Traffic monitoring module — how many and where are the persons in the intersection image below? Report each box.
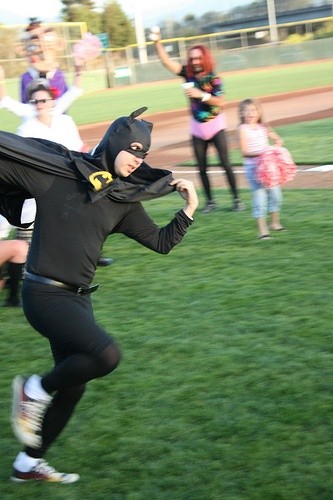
[0,48,115,309]
[150,26,244,213]
[0,108,199,483]
[238,99,285,241]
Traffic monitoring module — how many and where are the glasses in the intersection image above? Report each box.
[28,99,51,105]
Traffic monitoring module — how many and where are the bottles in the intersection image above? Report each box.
[148,25,159,40]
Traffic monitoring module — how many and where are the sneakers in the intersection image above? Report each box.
[10,372,49,449]
[9,457,80,485]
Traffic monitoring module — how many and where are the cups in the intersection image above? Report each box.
[182,81,194,91]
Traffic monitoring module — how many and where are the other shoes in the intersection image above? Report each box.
[271,223,283,231]
[232,200,245,212]
[256,231,271,240]
[200,202,218,214]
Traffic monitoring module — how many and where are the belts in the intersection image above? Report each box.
[21,271,100,295]
